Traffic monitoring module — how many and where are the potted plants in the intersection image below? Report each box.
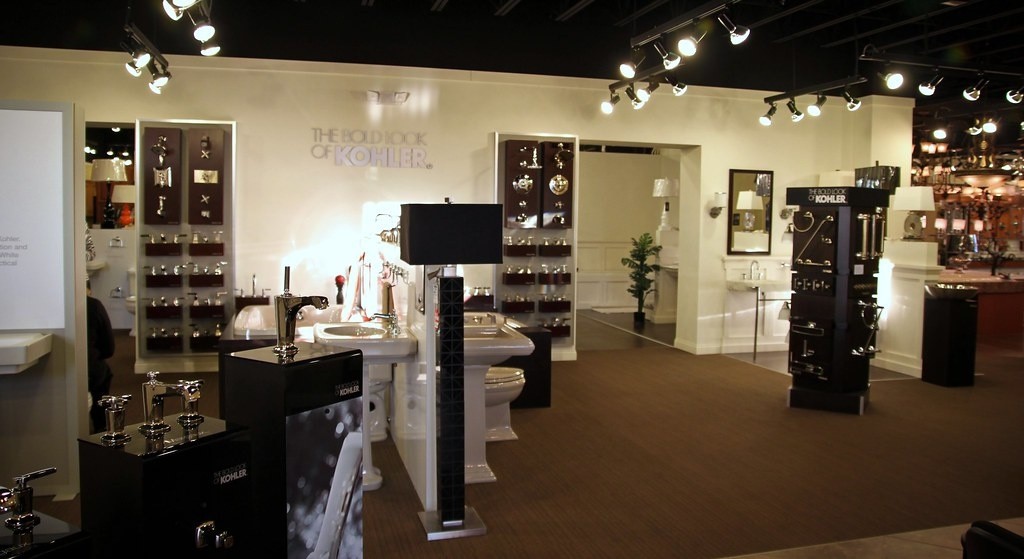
[621,233,662,329]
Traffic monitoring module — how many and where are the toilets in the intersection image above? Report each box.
[484,366,526,442]
[126,267,138,337]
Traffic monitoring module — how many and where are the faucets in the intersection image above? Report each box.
[749,259,759,280]
[503,234,574,330]
[138,228,225,341]
[251,272,259,298]
[141,368,201,431]
[273,264,332,360]
[369,309,400,335]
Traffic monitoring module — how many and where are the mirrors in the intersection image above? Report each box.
[727,169,773,255]
[86,120,136,232]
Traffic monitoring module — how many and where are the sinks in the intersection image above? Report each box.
[323,325,389,338]
[733,279,789,292]
[86,260,107,278]
[465,324,514,344]
[0,329,52,375]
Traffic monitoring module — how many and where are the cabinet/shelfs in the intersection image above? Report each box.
[133,119,236,375]
[489,132,579,361]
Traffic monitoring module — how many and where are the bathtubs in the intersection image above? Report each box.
[233,305,376,337]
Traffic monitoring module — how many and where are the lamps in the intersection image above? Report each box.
[600,60,690,114]
[366,90,410,106]
[777,302,791,320]
[163,0,220,56]
[910,140,1024,276]
[653,177,679,231]
[400,198,503,541]
[819,160,900,238]
[859,43,1024,104]
[620,1,751,79]
[756,174,771,232]
[152,136,218,217]
[912,107,1024,139]
[111,186,136,226]
[736,189,764,232]
[512,142,575,224]
[119,6,172,95]
[710,192,727,218]
[91,159,127,229]
[759,51,867,127]
[892,186,935,242]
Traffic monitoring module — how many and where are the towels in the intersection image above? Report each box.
[376,277,394,325]
[339,260,362,322]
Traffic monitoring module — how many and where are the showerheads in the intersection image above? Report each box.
[555,142,574,169]
[156,195,166,216]
[520,144,543,171]
